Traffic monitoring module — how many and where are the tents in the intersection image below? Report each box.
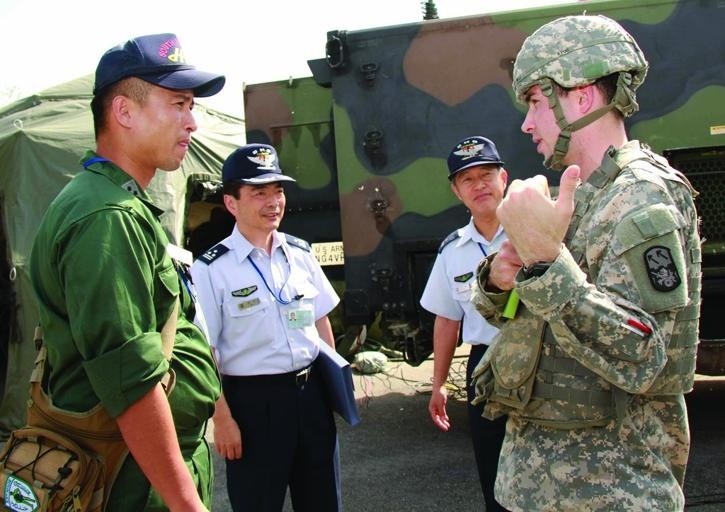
[0,72,248,442]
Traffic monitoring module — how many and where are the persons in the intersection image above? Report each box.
[419,136,511,511]
[27,33,226,511]
[187,144,343,511]
[470,14,704,511]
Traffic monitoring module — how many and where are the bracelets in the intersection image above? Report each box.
[429,377,447,383]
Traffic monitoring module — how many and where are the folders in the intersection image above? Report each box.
[318,337,362,426]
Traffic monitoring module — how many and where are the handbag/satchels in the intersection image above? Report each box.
[0,358,178,511]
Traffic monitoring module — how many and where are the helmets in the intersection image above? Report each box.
[511,13,649,105]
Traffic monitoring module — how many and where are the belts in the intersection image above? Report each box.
[293,367,313,389]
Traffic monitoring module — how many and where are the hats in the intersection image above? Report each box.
[94,34,226,98]
[446,136,504,179]
[222,143,297,186]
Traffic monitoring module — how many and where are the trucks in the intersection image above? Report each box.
[242,0,725,376]
